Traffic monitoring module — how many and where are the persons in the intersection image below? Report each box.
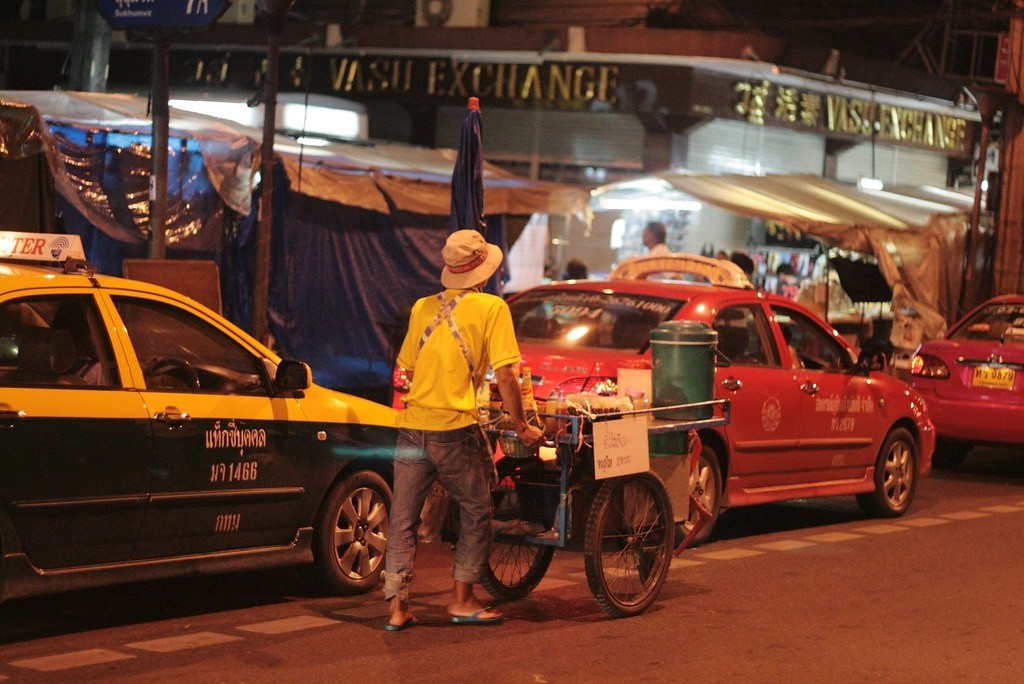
[776,266,801,299]
[643,221,685,280]
[564,257,588,280]
[51,300,107,386]
[757,323,805,369]
[378,230,549,631]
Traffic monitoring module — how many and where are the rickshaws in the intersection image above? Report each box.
[416,377,735,620]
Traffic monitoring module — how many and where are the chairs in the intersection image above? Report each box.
[716,325,749,358]
[612,311,651,349]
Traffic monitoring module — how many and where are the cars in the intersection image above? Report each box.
[0,227,412,604]
[438,277,939,552]
[909,291,1023,465]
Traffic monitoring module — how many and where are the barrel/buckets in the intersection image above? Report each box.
[648,319,732,419]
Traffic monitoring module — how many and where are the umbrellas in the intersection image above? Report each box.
[449,94,493,234]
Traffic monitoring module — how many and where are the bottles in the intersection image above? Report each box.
[514,368,651,443]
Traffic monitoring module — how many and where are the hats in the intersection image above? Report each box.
[440,229,504,289]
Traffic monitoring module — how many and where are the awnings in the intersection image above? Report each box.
[667,169,987,227]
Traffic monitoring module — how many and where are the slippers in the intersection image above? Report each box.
[384,613,418,631]
[452,606,506,622]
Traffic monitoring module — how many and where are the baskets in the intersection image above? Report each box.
[494,416,546,458]
[480,406,504,454]
